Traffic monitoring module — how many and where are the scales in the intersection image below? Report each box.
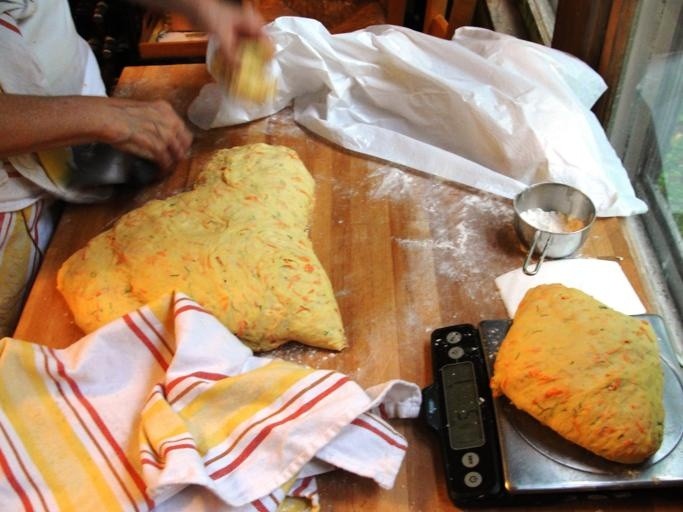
[419,313,683,509]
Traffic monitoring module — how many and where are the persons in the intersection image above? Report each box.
[0,0,279,343]
